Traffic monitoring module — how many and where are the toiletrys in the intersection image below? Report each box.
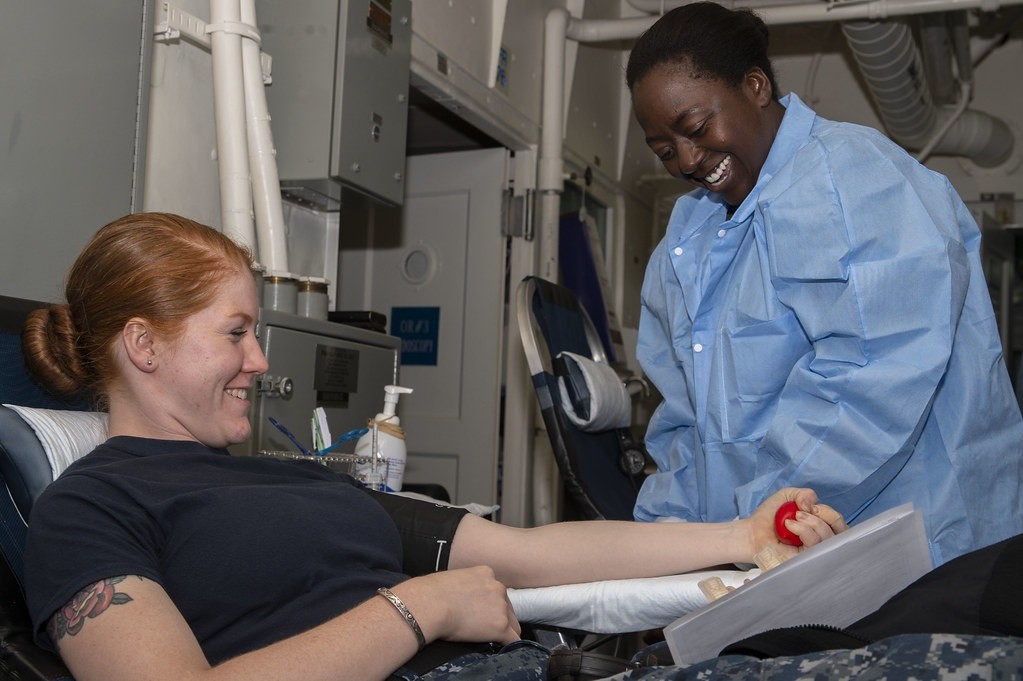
[350,384,414,493]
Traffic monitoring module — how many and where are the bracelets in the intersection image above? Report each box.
[377,588,425,651]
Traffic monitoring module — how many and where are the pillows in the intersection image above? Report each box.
[554,352,632,432]
[1,403,110,528]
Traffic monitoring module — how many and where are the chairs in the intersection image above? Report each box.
[515,276,652,521]
[0,296,114,681]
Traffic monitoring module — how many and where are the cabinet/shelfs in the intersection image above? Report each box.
[255,0,413,212]
[228,309,403,473]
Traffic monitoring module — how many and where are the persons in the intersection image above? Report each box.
[625,1,1023,659]
[21,213,850,681]
